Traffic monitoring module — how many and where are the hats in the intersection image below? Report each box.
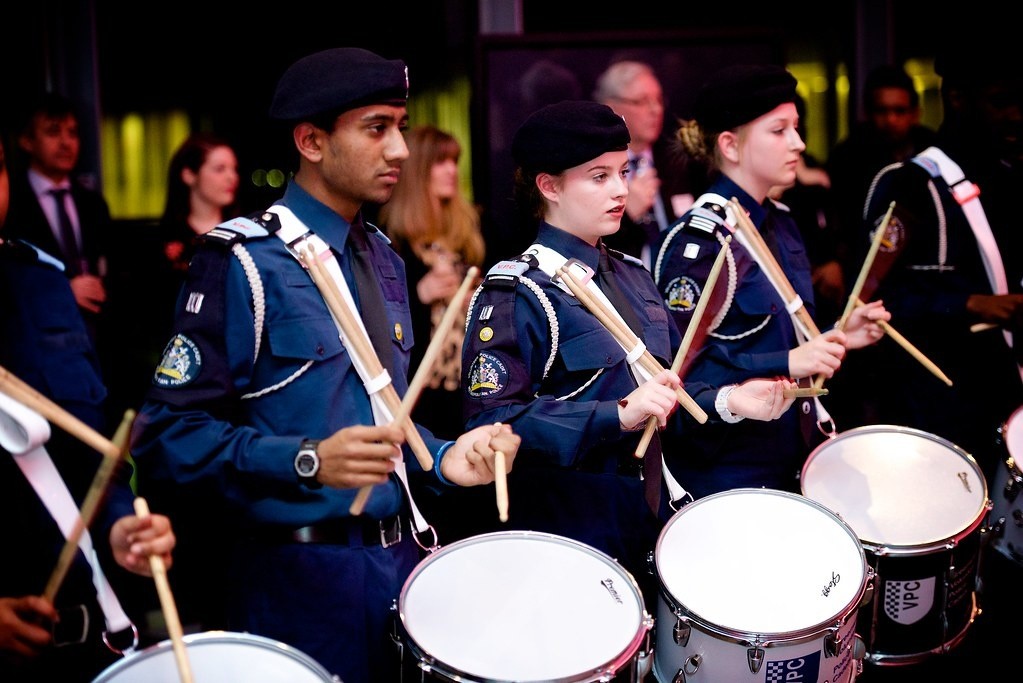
[694,63,798,133]
[511,100,630,178]
[271,48,410,132]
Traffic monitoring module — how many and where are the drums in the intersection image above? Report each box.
[985,403,1023,558]
[91,628,340,683]
[387,529,655,683]
[652,425,992,683]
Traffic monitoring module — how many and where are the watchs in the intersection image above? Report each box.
[294,439,321,490]
[715,384,744,423]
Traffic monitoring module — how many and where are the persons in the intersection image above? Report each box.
[649,60,890,495]
[597,60,696,277]
[782,67,940,297]
[13,96,128,366]
[128,136,238,382]
[862,13,1023,471]
[369,127,487,437]
[129,45,519,683]
[469,99,797,632]
[0,148,174,683]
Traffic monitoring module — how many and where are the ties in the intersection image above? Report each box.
[598,253,662,519]
[347,225,394,385]
[768,220,817,443]
[48,188,78,260]
[631,158,661,233]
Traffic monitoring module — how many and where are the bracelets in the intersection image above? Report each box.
[434,441,458,486]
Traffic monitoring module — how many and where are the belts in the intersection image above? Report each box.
[256,507,407,548]
[39,603,104,649]
